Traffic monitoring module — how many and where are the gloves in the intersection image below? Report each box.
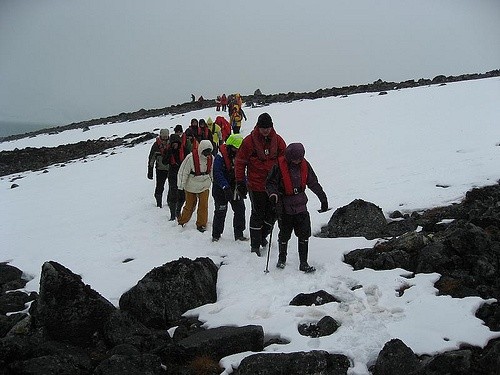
[237,181,247,199]
[318,203,328,212]
[269,196,277,207]
[178,189,184,194]
[148,166,153,179]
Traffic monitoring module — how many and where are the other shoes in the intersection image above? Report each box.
[212,236,219,242]
[197,226,206,232]
[235,236,249,241]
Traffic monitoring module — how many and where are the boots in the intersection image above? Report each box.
[298,238,316,271]
[277,240,288,268]
[260,220,272,247]
[249,227,261,257]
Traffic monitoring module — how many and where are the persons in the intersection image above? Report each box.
[191,94,195,101]
[215,93,247,141]
[148,118,222,223]
[177,140,215,231]
[198,96,204,101]
[265,143,329,271]
[235,113,286,256]
[211,134,248,241]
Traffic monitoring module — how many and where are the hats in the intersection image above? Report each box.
[207,117,213,124]
[258,112,273,128]
